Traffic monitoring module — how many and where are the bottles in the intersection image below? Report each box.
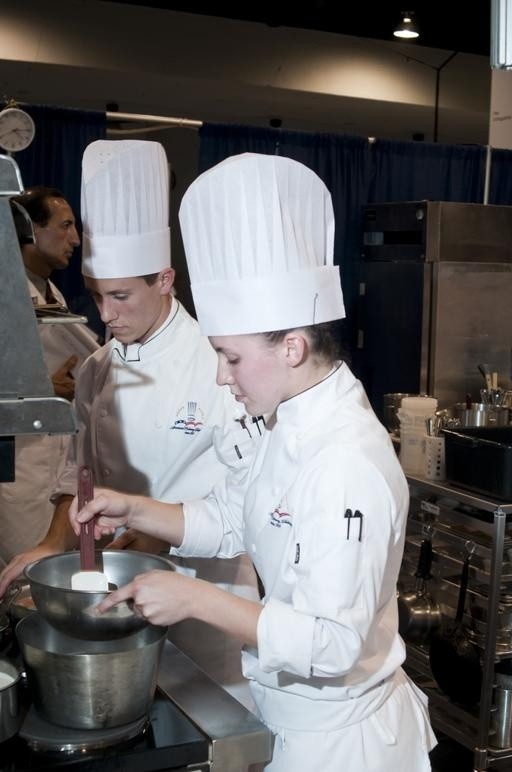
[398,397,438,476]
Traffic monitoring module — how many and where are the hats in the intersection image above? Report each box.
[78,140,170,278]
[179,152,348,336]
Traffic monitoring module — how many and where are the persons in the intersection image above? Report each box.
[1,269,264,728]
[1,185,102,597]
[64,321,442,770]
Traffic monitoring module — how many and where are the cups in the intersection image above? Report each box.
[488,687,511,749]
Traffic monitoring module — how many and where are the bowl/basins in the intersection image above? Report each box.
[455,403,508,426]
[384,393,440,437]
[25,547,175,640]
[0,586,170,741]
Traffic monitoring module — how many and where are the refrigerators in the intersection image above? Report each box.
[357,202,510,436]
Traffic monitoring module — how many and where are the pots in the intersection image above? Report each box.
[397,539,442,644]
[428,559,483,708]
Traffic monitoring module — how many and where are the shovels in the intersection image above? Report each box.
[71,465,109,591]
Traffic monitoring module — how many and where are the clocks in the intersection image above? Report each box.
[0,108,35,153]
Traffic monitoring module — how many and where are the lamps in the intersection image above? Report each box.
[393,10,420,38]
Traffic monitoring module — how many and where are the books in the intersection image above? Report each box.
[38,322,103,381]
